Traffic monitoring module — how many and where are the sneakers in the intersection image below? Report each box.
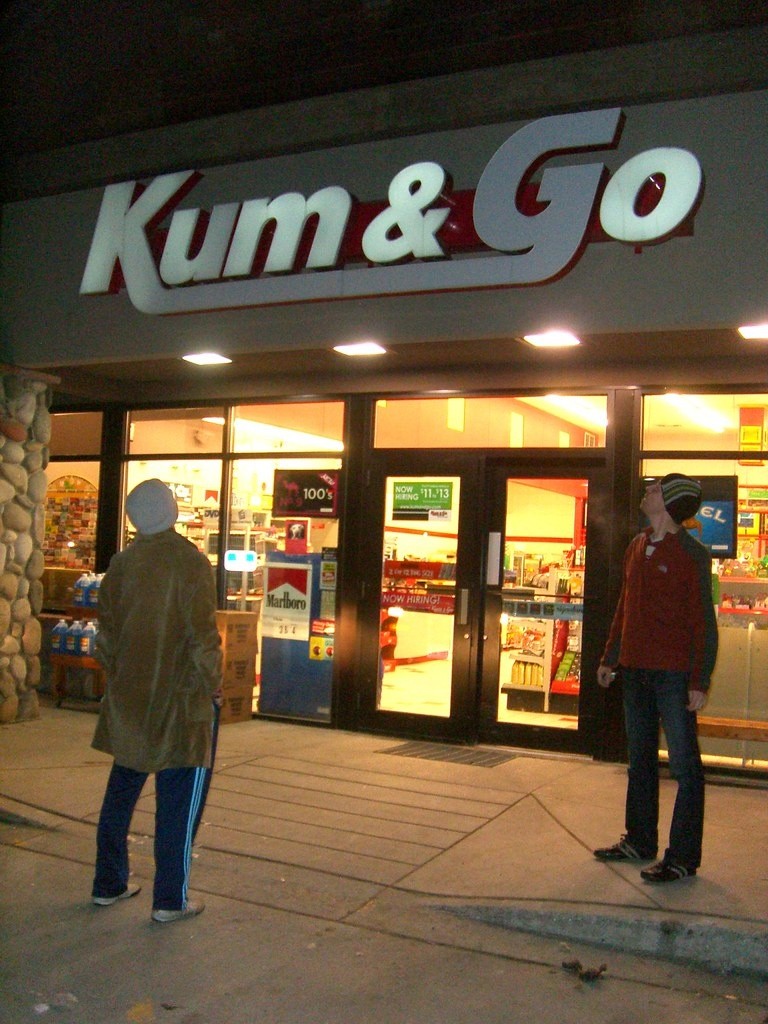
[594,834,658,860]
[641,848,697,882]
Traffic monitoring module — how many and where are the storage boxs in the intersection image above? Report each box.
[214,609,258,726]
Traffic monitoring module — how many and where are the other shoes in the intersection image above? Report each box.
[93,884,141,905]
[151,900,205,922]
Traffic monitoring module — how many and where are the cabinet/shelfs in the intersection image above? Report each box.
[204,531,265,603]
[500,565,555,712]
[718,576,768,618]
[50,606,103,708]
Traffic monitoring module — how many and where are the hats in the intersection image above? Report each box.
[661,473,703,525]
[126,479,178,535]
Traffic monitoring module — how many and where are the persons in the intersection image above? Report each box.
[593,473,719,885]
[91,478,224,922]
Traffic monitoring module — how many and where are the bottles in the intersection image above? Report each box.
[74,572,104,607]
[511,661,543,686]
[52,619,99,655]
